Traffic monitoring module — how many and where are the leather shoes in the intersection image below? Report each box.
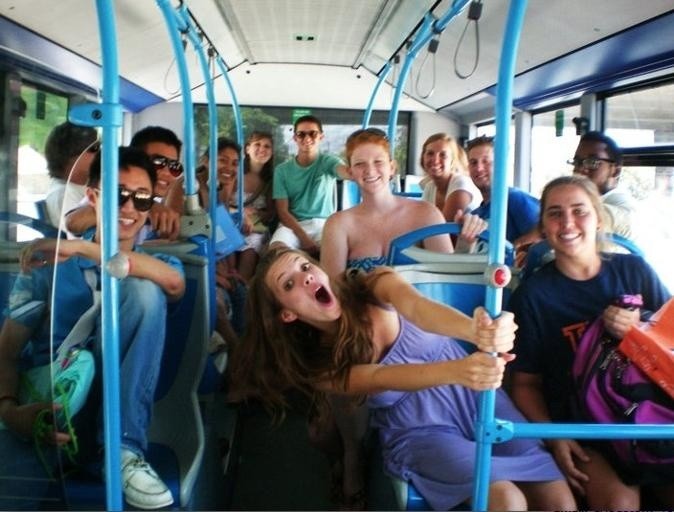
[97,446,174,510]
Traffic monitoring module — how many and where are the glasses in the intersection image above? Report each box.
[148,153,185,178]
[345,128,387,141]
[568,156,614,170]
[294,130,321,139]
[94,186,155,213]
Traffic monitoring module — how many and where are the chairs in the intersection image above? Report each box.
[1,175,245,512]
[341,175,644,512]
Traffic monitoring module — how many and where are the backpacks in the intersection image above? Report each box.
[571,299,674,487]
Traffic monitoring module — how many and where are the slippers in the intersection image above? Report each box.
[326,470,370,510]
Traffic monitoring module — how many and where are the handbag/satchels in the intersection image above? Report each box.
[246,210,270,234]
[614,296,674,402]
